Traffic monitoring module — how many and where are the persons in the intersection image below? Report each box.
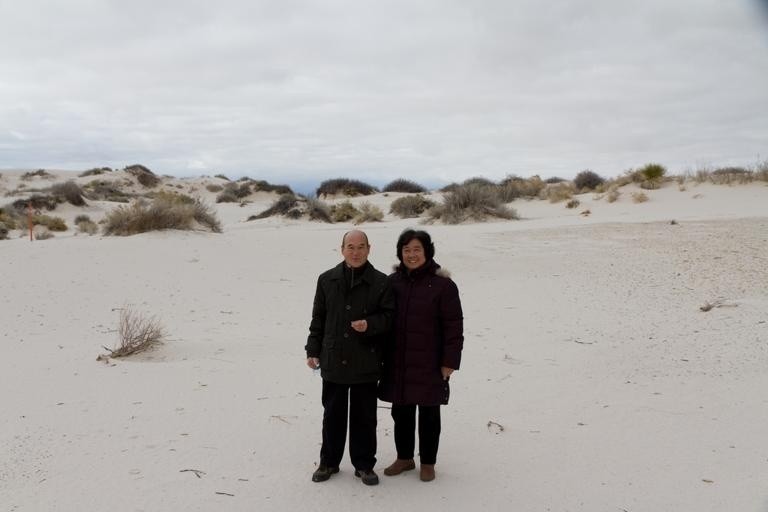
[306,232,397,484]
[381,228,463,481]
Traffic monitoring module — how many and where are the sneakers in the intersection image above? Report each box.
[355,469,378,484]
[420,463,435,481]
[312,466,339,482]
[384,459,415,475]
